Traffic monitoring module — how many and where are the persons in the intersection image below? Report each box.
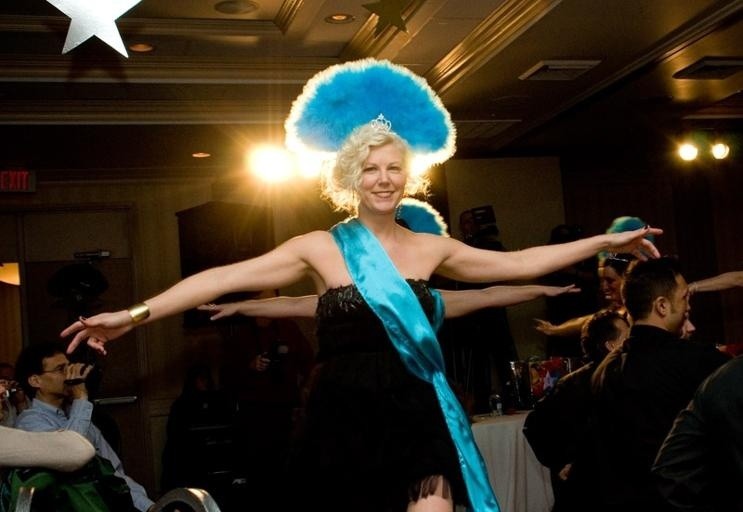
[451,210,522,391]
[220,289,315,512]
[199,219,581,512]
[58,119,664,512]
[13,343,156,512]
[159,360,221,512]
[0,362,29,427]
[0,425,98,472]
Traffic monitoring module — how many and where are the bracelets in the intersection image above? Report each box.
[127,301,149,323]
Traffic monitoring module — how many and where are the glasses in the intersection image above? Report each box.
[41,364,68,374]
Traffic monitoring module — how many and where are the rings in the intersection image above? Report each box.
[208,302,216,311]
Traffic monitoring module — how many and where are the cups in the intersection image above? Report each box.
[63,359,104,390]
